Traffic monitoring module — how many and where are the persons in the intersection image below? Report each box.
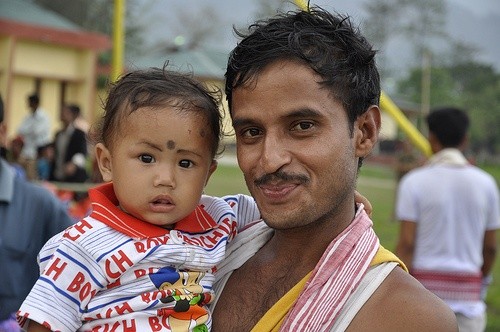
[394,109,500,332]
[15,60,374,332]
[0,95,89,323]
[208,0,459,332]
